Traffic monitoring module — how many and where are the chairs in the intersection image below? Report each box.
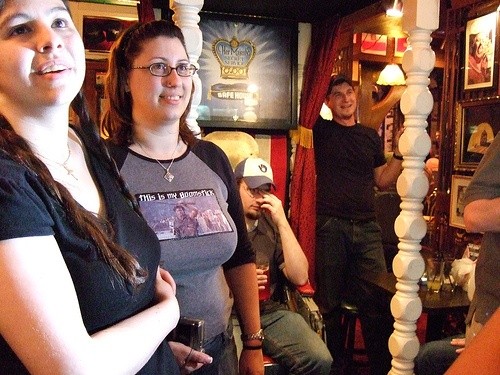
[171,263,324,375]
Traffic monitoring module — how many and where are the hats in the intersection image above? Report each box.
[330,73,353,85]
[235,157,277,191]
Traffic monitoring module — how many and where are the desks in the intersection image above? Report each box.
[380,273,470,342]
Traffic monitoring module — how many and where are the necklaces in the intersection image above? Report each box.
[133,137,186,181]
[28,141,78,181]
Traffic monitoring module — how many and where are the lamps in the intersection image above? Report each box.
[376,63,407,87]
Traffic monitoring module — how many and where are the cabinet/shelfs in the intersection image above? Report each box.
[434,3,500,259]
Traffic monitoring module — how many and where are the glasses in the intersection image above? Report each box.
[132,63,198,77]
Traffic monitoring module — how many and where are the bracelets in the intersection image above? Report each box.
[242,342,264,350]
[393,152,403,161]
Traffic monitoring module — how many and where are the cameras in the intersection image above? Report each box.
[170,317,206,352]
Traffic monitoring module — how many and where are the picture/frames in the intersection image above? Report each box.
[451,95,499,177]
[462,9,500,93]
[448,175,472,230]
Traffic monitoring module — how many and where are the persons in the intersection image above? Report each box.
[412,331,469,375]
[85,19,268,375]
[462,122,499,357]
[293,76,409,375]
[0,0,188,375]
[208,155,334,375]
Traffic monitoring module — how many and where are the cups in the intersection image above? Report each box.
[256,259,271,301]
[426,259,445,293]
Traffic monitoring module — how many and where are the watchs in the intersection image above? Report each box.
[241,329,265,342]
